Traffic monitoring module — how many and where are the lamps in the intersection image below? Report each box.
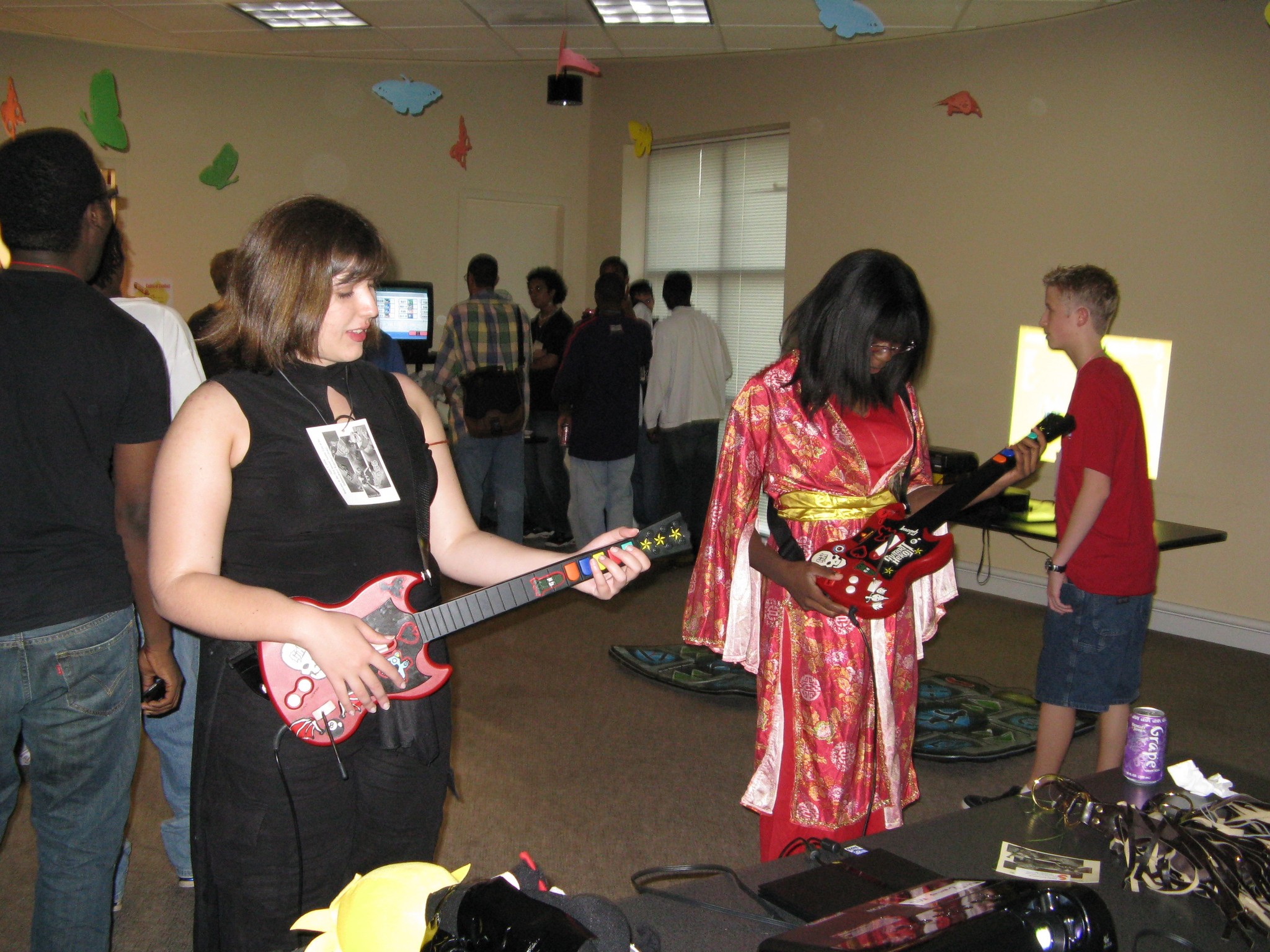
[546,67,583,107]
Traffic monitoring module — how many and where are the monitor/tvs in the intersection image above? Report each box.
[372,279,434,355]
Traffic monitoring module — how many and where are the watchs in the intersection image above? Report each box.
[1045,558,1066,573]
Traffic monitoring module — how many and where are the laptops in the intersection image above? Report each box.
[758,848,949,923]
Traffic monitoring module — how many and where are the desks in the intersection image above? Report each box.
[945,493,1231,553]
[404,350,441,372]
[626,749,1270,952]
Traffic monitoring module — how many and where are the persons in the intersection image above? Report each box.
[361,326,409,376]
[86,224,206,910]
[150,194,651,952]
[961,263,1159,809]
[682,249,1047,862]
[643,270,733,560]
[187,249,244,381]
[431,253,533,545]
[522,266,575,547]
[0,128,172,952]
[553,256,655,550]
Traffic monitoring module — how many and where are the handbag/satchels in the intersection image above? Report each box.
[461,362,525,437]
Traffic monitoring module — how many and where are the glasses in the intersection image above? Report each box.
[464,272,467,281]
[528,286,549,295]
[870,339,915,355]
[77,184,120,219]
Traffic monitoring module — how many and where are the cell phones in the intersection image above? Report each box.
[143,679,166,702]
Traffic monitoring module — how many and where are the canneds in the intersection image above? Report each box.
[1121,707,1167,785]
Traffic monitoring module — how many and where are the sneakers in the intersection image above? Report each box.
[523,527,555,540]
[960,785,1022,810]
[545,530,576,549]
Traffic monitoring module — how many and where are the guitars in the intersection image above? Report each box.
[811,414,1075,621]
[257,511,691,747]
